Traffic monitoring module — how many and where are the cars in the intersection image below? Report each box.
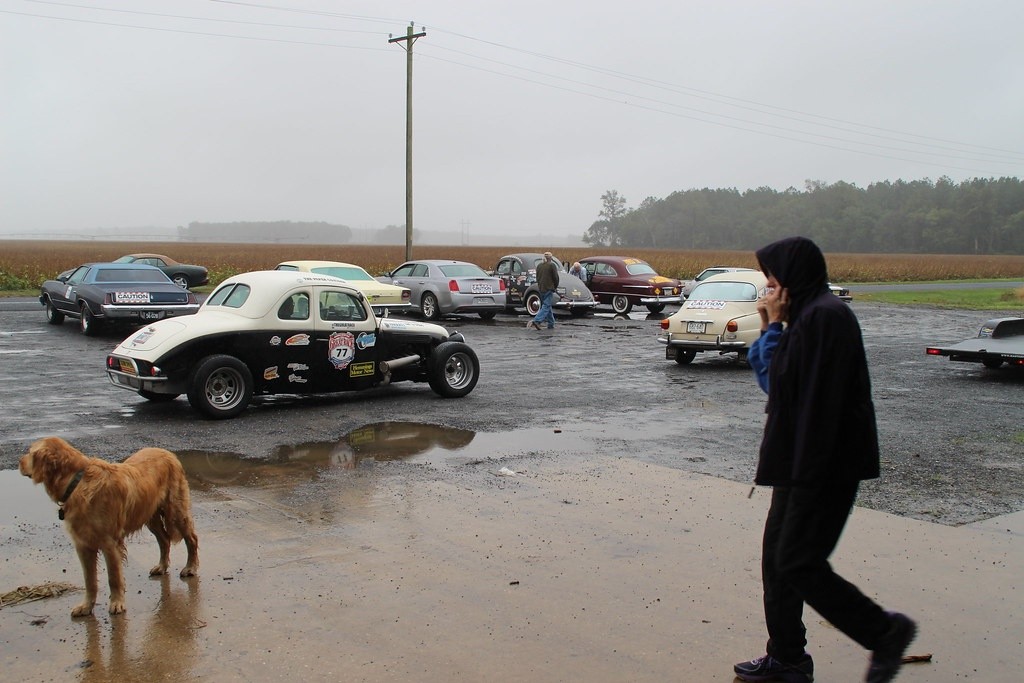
[486,252,600,318]
[677,267,758,299]
[371,260,507,321]
[273,260,412,319]
[578,256,684,314]
[105,270,480,421]
[659,272,788,369]
[56,253,210,290]
[38,261,201,337]
[828,283,852,303]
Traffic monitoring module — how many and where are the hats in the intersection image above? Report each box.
[544,252,552,257]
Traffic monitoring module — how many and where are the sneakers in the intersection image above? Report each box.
[865,611,918,683]
[734,650,814,683]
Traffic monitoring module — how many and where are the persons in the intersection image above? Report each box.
[732,235,919,683]
[568,262,587,284]
[531,252,559,331]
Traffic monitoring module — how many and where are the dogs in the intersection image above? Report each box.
[17,436,199,618]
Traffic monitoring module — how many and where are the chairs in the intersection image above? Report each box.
[298,297,311,318]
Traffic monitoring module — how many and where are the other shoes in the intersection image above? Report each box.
[547,326,554,329]
[532,321,541,330]
